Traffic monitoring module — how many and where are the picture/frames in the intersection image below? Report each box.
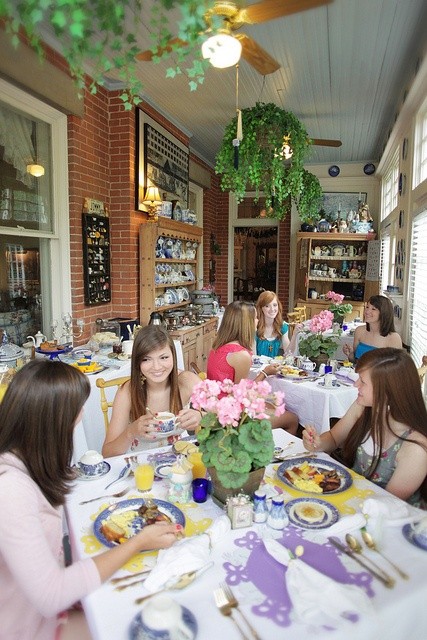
[323,192,367,216]
[135,107,190,213]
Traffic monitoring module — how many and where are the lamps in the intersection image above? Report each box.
[26,163,45,177]
[200,1,242,68]
[142,186,163,222]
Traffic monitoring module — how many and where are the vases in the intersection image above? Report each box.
[332,315,346,328]
[301,221,314,232]
[309,354,329,372]
[207,467,266,510]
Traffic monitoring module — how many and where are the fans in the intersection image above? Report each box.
[137,0,338,76]
[310,138,341,147]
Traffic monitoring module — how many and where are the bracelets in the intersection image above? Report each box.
[197,409,202,418]
[260,370,267,378]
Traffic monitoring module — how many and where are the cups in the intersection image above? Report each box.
[128,599,198,640]
[134,458,155,494]
[274,447,284,459]
[350,328,355,335]
[191,478,208,503]
[155,236,199,284]
[333,323,339,333]
[77,450,104,476]
[112,340,122,355]
[309,262,336,279]
[413,517,427,550]
[152,412,181,432]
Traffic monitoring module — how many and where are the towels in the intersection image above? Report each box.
[319,497,426,536]
[144,515,232,590]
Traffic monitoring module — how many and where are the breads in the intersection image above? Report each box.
[285,469,300,483]
[293,467,308,480]
[100,520,123,540]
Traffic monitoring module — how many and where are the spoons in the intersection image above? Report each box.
[345,534,396,584]
[362,528,409,580]
[274,441,295,455]
[105,463,132,490]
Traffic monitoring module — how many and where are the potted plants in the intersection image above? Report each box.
[215,102,322,221]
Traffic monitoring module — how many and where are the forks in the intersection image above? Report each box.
[81,486,132,504]
[213,588,249,640]
[219,581,261,640]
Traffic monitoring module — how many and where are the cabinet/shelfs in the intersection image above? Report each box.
[139,216,203,327]
[82,213,112,306]
[234,246,243,272]
[181,320,218,375]
[297,301,368,322]
[297,232,376,301]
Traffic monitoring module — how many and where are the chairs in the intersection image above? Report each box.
[234,277,240,300]
[402,341,410,354]
[418,356,427,409]
[96,375,132,436]
[293,306,306,322]
[237,278,254,302]
[287,312,302,341]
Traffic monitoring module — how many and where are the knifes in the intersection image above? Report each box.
[327,536,393,589]
[61,512,72,567]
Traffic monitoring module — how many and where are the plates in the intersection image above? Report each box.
[76,359,92,366]
[92,498,185,552]
[276,458,353,495]
[72,461,112,481]
[284,497,340,531]
[84,360,109,375]
[346,333,354,337]
[402,522,427,552]
[147,428,176,438]
[128,605,198,640]
[188,452,206,480]
[155,463,172,479]
[318,381,340,389]
[72,349,95,359]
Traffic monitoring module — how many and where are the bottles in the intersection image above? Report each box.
[267,495,288,530]
[324,366,333,387]
[276,370,319,379]
[252,490,269,523]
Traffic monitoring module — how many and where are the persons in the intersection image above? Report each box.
[206,300,298,436]
[0,359,185,639]
[301,344,426,510]
[342,295,402,366]
[252,290,304,360]
[101,325,208,461]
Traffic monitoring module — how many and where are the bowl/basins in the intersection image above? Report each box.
[324,377,337,386]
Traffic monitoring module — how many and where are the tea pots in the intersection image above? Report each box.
[27,331,47,358]
[300,357,316,372]
[148,312,162,326]
[155,286,191,307]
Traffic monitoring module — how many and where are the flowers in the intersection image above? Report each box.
[296,310,342,357]
[326,291,353,322]
[191,379,285,489]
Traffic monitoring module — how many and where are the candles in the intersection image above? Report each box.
[339,200,341,211]
[359,192,361,200]
